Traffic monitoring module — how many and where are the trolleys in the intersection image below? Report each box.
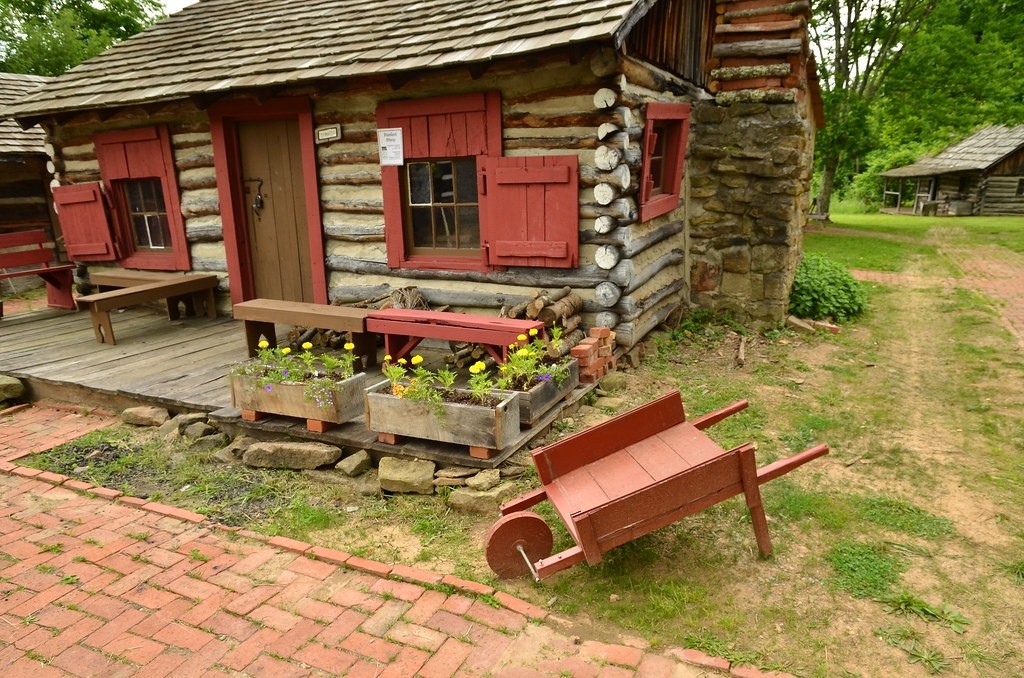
[484,389,830,588]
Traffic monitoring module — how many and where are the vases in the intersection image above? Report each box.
[229,366,367,423]
[363,379,520,450]
[490,356,580,425]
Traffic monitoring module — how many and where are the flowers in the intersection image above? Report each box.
[497,320,571,389]
[382,354,492,429]
[235,335,362,408]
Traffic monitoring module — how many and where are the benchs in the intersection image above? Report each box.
[0,229,77,310]
[366,308,544,377]
[75,274,220,345]
[89,270,193,321]
[233,298,375,373]
